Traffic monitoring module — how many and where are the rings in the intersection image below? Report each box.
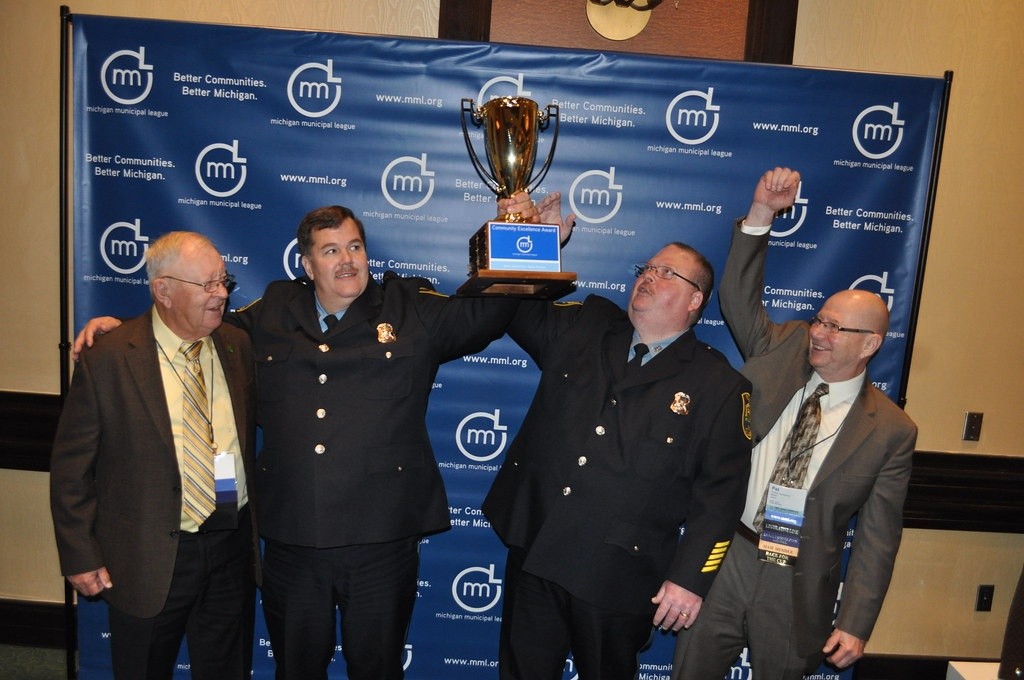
[681,613,689,618]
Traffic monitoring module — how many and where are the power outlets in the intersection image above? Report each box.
[976,585,995,612]
[962,411,984,441]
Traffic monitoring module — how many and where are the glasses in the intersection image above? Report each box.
[809,315,875,334]
[634,262,700,291]
[161,273,235,292]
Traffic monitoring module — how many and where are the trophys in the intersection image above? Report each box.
[460,96,576,297]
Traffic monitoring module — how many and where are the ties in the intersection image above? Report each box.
[322,314,338,328]
[178,340,217,526]
[629,342,650,368]
[753,382,830,533]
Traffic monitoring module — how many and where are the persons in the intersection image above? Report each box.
[70,195,541,680]
[670,167,918,680]
[480,192,752,680]
[50,231,258,680]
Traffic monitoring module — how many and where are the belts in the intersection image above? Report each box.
[737,520,760,548]
